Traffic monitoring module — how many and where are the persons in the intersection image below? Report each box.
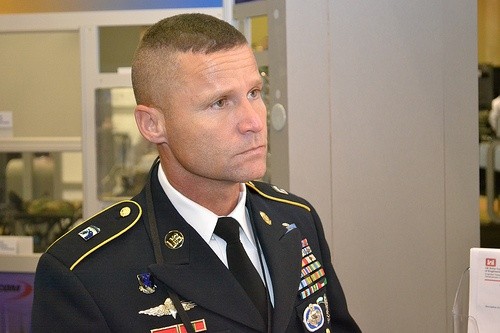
[31,13,363,333]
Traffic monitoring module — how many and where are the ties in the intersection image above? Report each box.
[213,217,274,333]
